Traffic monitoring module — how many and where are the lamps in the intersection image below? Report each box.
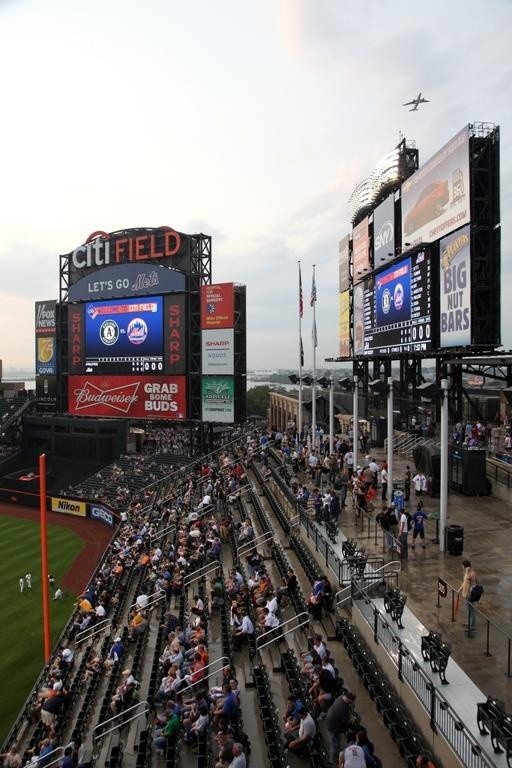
[288,374,443,404]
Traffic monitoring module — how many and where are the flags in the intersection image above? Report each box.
[310,276,317,307]
[299,269,304,318]
[300,338,304,366]
[312,321,317,347]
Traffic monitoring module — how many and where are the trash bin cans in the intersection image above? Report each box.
[445,525,463,556]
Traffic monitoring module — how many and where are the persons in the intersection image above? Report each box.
[1,409,511,768]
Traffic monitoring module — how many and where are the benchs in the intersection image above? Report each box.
[0,397,26,426]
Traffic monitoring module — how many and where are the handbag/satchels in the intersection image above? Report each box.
[468,585,483,602]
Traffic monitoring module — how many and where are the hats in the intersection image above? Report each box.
[122,669,131,675]
[162,709,173,717]
[296,704,305,714]
